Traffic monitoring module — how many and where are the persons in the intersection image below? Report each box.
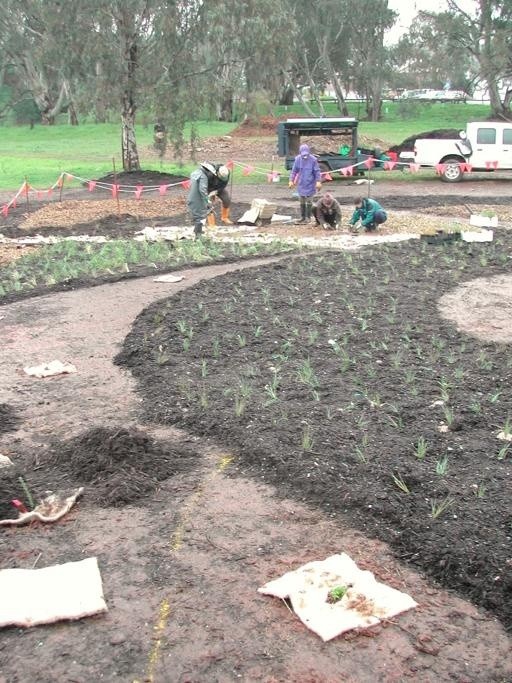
[187,160,217,243]
[348,197,387,232]
[206,164,234,227]
[288,143,322,225]
[311,193,342,231]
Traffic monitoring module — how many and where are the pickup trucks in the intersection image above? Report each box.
[413,120,512,183]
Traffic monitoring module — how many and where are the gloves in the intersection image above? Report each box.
[288,181,293,189]
[315,182,322,191]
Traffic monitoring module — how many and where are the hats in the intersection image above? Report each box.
[200,163,217,177]
[217,165,229,181]
[323,192,333,207]
[299,144,309,156]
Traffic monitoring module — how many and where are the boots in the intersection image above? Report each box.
[221,207,234,225]
[194,223,205,239]
[296,204,306,222]
[193,219,205,234]
[207,213,217,231]
[304,203,312,223]
